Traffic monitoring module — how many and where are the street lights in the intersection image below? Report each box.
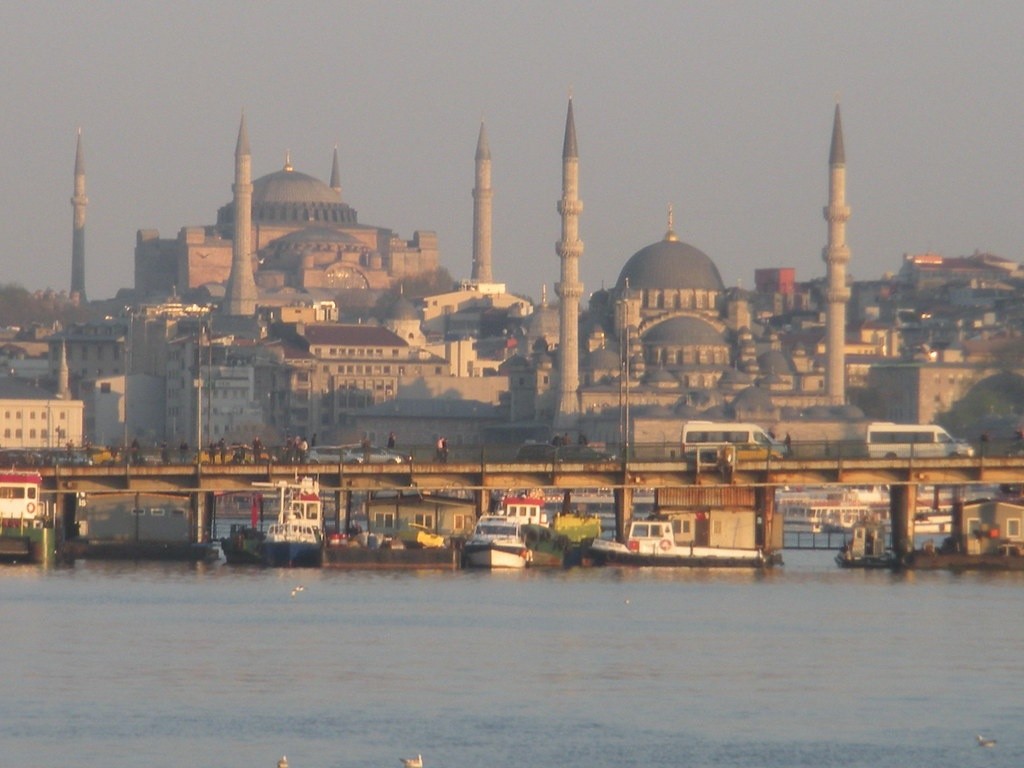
[183,303,208,488]
[617,277,632,483]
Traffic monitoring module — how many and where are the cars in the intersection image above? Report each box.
[512,440,617,465]
[192,441,415,467]
[0,446,114,468]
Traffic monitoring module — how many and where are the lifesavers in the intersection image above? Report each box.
[659,539,672,551]
[27,502,35,513]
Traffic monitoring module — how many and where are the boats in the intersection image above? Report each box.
[461,507,528,569]
[1,470,45,558]
[250,475,325,568]
[219,523,264,562]
[833,520,891,568]
[808,485,954,534]
[580,516,767,568]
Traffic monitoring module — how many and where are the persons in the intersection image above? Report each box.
[432,436,449,464]
[361,435,370,465]
[160,440,169,465]
[210,432,318,465]
[84,433,124,466]
[180,438,188,465]
[388,432,395,449]
[552,430,587,446]
[785,432,791,452]
[768,428,776,439]
[131,438,139,465]
[65,439,75,464]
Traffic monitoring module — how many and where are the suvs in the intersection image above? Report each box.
[722,441,784,462]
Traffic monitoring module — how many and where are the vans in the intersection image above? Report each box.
[863,420,975,461]
[680,418,791,460]
